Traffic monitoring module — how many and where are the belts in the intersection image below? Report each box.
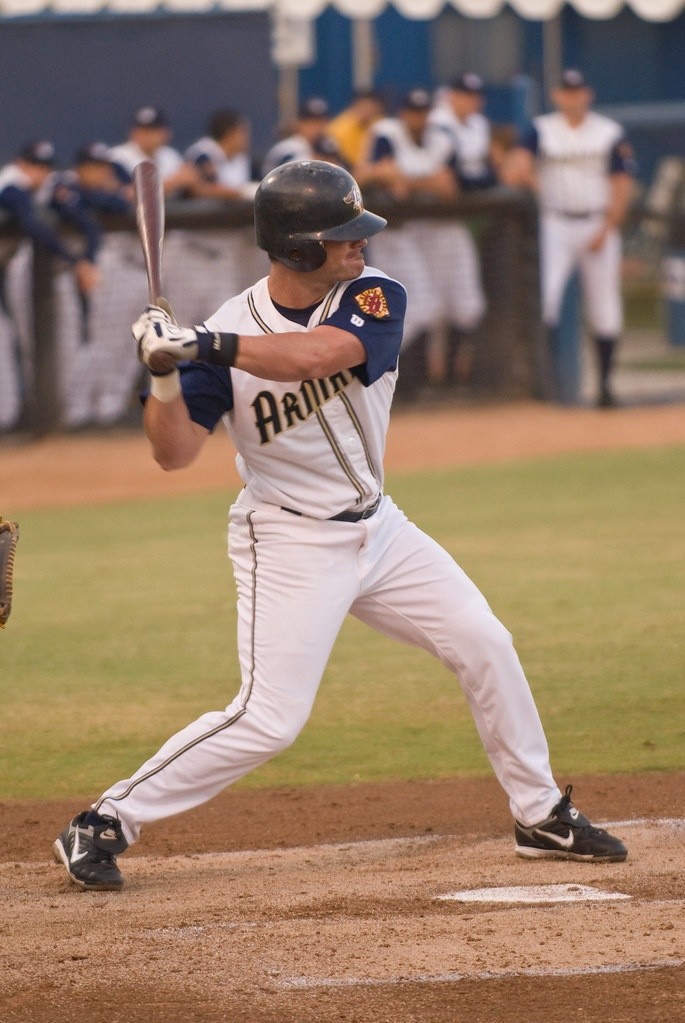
[280,493,381,523]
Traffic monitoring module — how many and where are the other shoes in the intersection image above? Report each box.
[413,324,465,390]
[590,336,619,408]
[531,321,559,400]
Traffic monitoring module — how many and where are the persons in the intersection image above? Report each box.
[499,67,646,410]
[49,162,630,894]
[0,71,531,439]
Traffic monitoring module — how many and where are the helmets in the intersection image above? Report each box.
[252,160,388,274]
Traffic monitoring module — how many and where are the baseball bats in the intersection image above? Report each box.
[132,159,176,374]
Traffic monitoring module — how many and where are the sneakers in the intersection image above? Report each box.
[51,810,128,890]
[514,784,628,863]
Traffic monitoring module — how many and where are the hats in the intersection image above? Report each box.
[554,67,589,90]
[17,137,56,163]
[295,96,328,116]
[451,70,484,92]
[73,138,116,162]
[131,105,170,125]
[396,88,431,108]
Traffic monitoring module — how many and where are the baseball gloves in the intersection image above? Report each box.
[0,516,18,628]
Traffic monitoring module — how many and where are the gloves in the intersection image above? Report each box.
[129,297,183,403]
[136,320,238,370]
